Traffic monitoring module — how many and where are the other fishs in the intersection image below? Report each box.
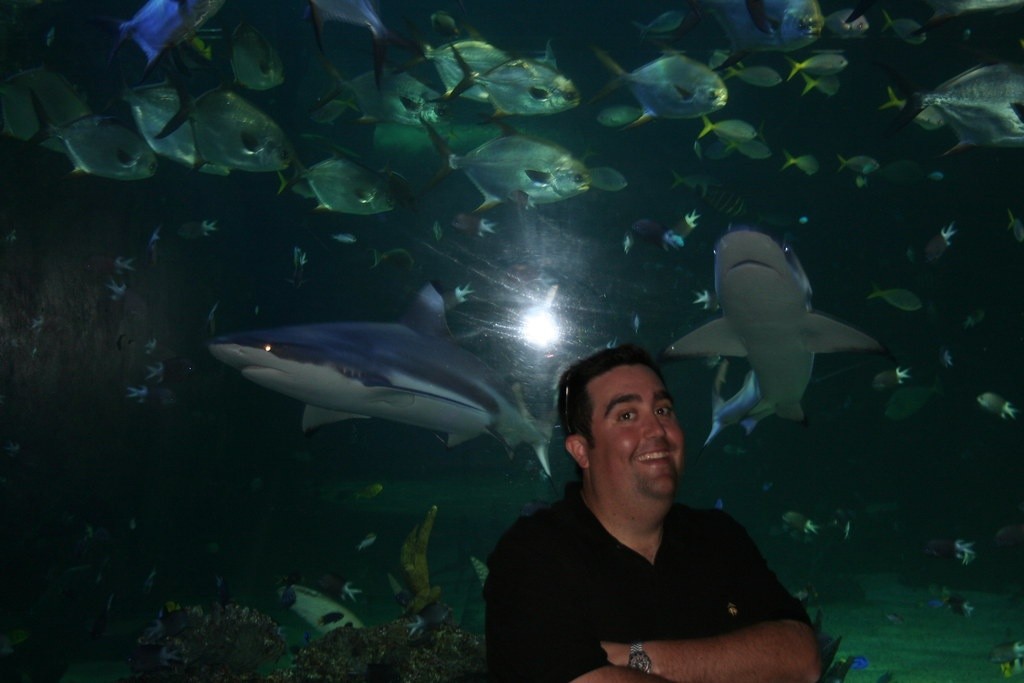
[0,0,1023,244]
[0,246,486,671]
[632,259,1024,683]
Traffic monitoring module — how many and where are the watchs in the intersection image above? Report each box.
[627,642,651,675]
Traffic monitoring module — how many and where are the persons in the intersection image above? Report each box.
[482,346,821,683]
